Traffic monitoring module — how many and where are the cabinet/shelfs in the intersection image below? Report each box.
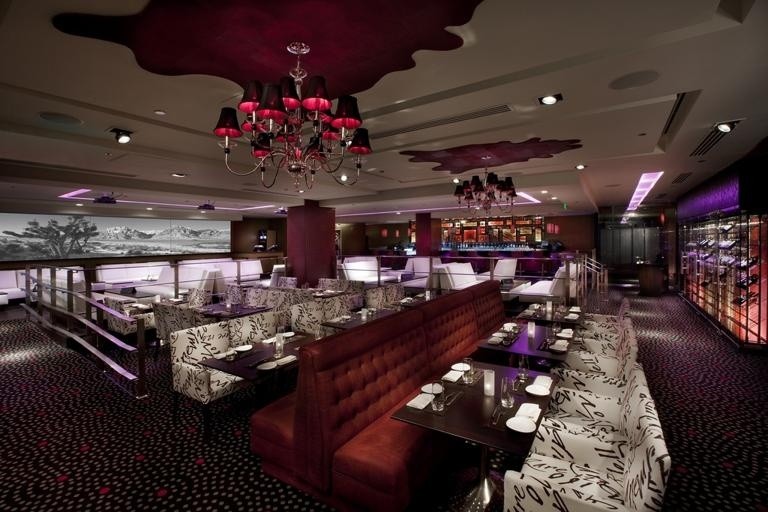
[673,211,766,353]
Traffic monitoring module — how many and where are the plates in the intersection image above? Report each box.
[422,382,441,393]
[552,331,572,350]
[280,332,295,338]
[527,384,551,396]
[237,343,277,371]
[450,362,468,373]
[492,321,515,339]
[506,417,536,433]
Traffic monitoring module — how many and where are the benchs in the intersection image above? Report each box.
[253,279,511,512]
[0,254,578,309]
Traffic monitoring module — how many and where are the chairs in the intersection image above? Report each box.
[503,296,672,512]
[104,297,351,403]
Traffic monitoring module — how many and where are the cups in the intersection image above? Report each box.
[430,380,445,413]
[501,378,515,409]
[515,357,529,383]
[462,357,475,385]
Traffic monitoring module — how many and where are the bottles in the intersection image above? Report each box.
[698,223,755,303]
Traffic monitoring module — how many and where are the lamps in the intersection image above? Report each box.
[195,201,217,213]
[201,41,374,193]
[113,130,131,145]
[454,149,516,214]
[92,195,119,206]
